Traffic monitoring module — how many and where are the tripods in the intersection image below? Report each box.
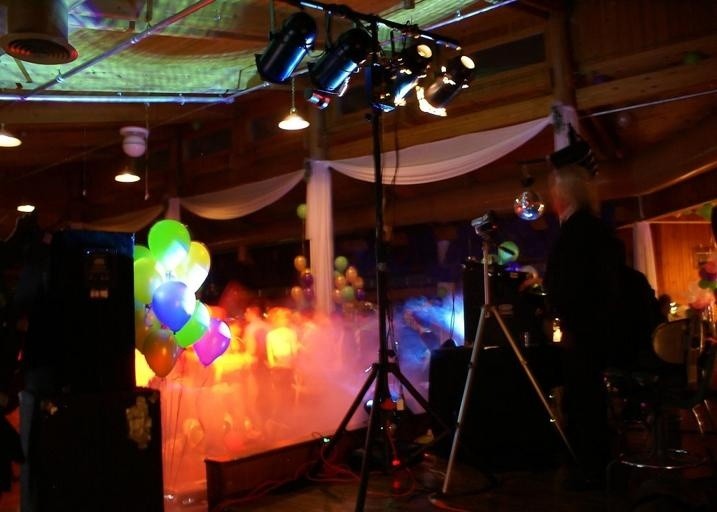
[441,238,574,499]
[321,22,453,512]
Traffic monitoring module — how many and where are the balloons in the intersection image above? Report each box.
[697,201,716,289]
[297,203,308,219]
[481,242,523,273]
[618,109,638,136]
[129,216,231,381]
[290,251,375,316]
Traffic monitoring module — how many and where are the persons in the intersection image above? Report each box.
[515,168,717,485]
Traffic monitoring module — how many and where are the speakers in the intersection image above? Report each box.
[21,389,163,512]
[18,228,134,401]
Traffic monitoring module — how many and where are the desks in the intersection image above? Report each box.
[426,342,576,472]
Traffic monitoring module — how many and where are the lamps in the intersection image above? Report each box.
[118,126,150,159]
[115,154,140,183]
[513,176,546,221]
[0,0,79,65]
[248,0,479,129]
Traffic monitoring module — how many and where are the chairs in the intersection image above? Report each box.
[596,315,717,512]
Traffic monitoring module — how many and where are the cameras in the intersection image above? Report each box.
[471,210,498,238]
